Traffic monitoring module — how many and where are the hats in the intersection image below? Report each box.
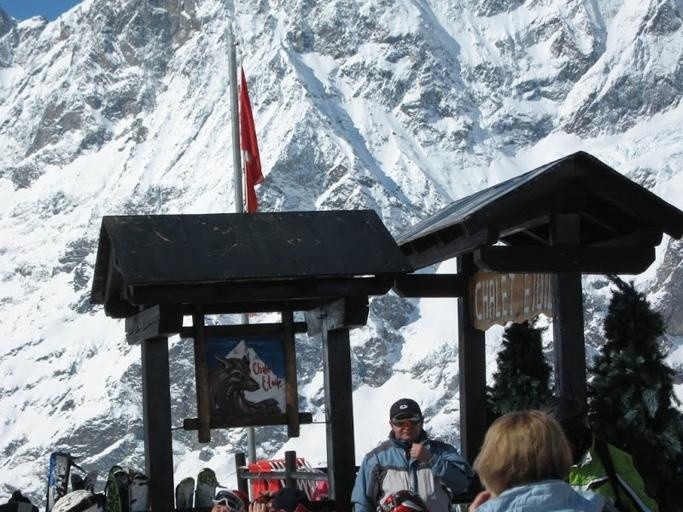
[266,487,312,509]
[390,399,421,423]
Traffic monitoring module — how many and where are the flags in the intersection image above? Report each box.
[238,66,262,212]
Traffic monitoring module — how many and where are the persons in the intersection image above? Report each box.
[204,488,430,512]
[538,397,660,512]
[351,398,473,512]
[468,408,619,511]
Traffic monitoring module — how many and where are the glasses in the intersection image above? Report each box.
[212,491,244,512]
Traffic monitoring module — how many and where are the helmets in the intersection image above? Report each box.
[51,489,106,512]
[376,490,429,512]
